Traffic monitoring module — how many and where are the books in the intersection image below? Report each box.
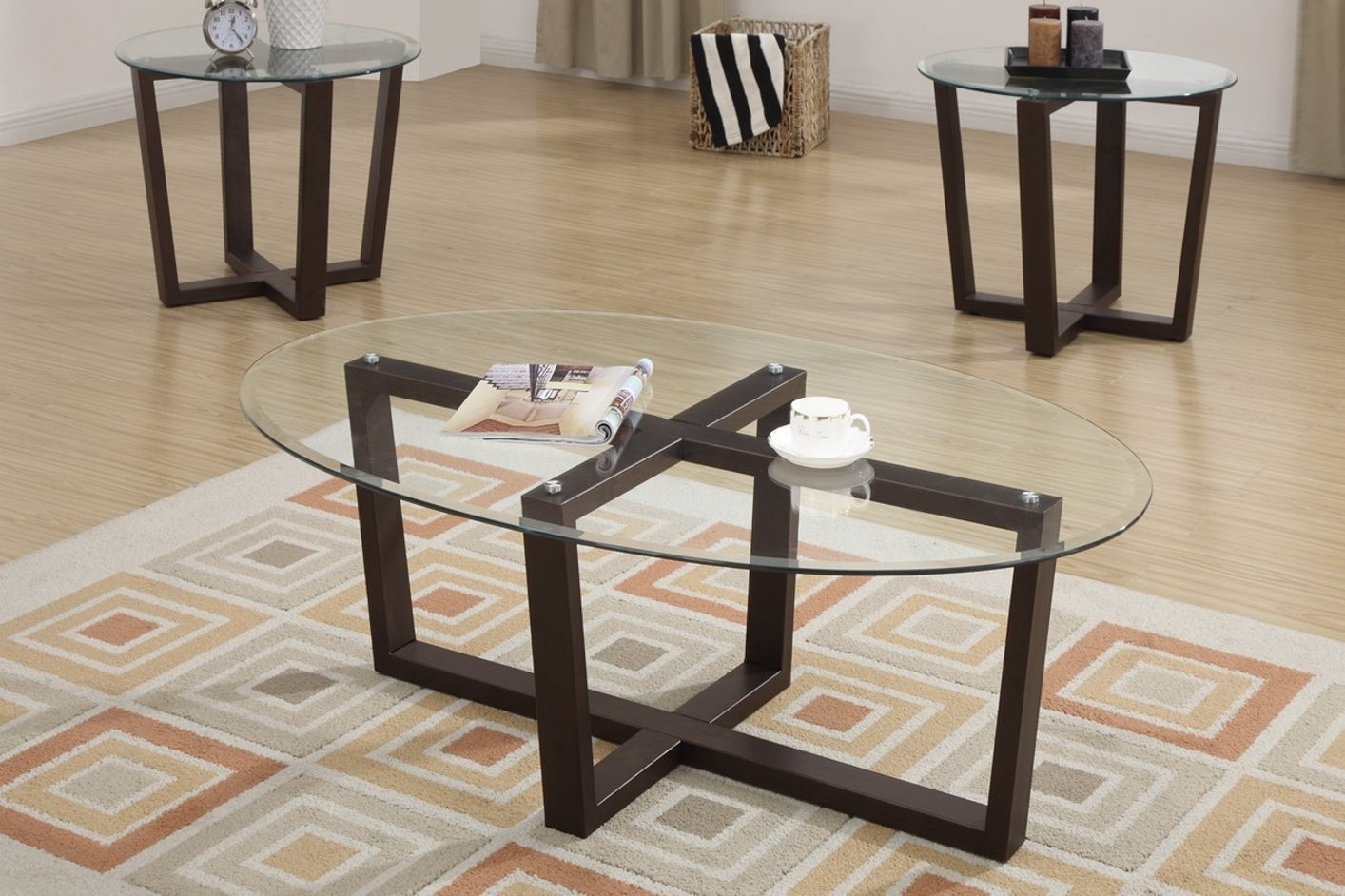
[441,358,654,448]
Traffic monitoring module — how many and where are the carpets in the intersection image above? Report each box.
[0,403,1344,895]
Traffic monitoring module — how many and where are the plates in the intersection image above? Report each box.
[769,425,874,470]
[768,456,874,490]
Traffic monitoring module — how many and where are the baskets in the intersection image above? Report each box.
[687,18,830,158]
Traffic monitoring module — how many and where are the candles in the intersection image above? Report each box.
[1070,15,1105,67]
[1029,0,1061,19]
[1029,16,1061,66]
[1066,1,1099,66]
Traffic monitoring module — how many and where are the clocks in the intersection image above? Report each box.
[205,56,256,78]
[202,0,257,61]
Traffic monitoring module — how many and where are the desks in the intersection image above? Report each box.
[916,46,1238,355]
[237,308,1153,864]
[116,21,425,321]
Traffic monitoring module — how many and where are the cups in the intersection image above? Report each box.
[790,396,870,457]
[266,0,327,49]
[267,47,325,76]
[791,484,871,517]
